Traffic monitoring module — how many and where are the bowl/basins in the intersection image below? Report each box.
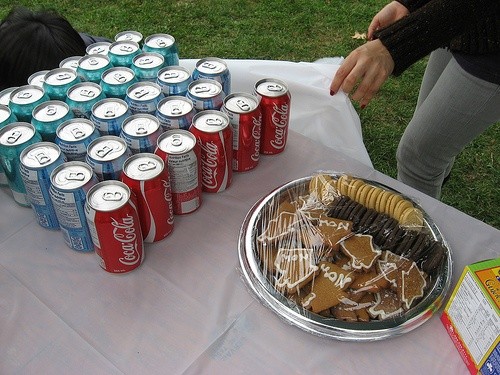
[236,174,454,343]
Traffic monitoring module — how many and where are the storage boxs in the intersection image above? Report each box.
[441,257,500,375]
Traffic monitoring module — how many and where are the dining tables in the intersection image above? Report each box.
[0,128,500,375]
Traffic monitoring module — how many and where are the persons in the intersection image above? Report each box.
[330,0,500,202]
[0,7,114,88]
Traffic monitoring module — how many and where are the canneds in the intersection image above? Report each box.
[220,93,262,173]
[0,31,231,273]
[253,78,292,156]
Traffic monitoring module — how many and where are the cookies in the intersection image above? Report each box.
[257,175,447,323]
